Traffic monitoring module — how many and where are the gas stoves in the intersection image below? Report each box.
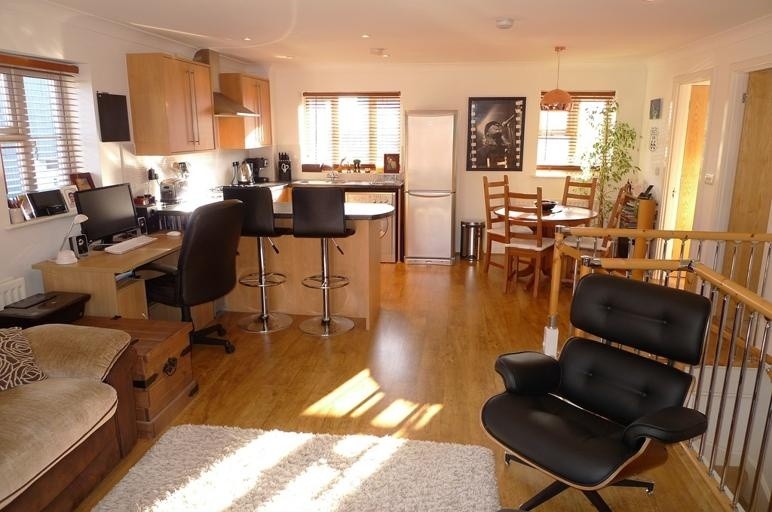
[210,185,223,199]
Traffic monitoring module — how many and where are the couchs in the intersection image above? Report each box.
[3,320,145,511]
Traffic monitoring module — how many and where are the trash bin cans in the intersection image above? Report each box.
[460,218,484,261]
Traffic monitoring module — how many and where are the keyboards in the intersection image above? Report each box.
[104,234,158,255]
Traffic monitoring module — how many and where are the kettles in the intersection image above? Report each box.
[236,161,253,185]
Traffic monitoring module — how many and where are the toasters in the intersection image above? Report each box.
[159,177,188,203]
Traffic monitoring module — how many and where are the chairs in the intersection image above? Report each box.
[481,175,528,279]
[291,182,364,338]
[223,184,295,333]
[560,175,600,261]
[477,274,710,512]
[502,184,556,299]
[128,198,244,353]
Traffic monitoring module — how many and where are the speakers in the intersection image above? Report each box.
[138,216,148,235]
[97,90,131,143]
[68,233,89,257]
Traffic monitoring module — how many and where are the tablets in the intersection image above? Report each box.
[25,189,70,219]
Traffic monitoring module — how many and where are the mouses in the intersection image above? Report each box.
[166,230,182,236]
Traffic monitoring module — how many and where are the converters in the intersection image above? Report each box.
[148,168,156,180]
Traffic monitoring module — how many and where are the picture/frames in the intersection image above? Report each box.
[465,97,525,172]
[650,101,663,120]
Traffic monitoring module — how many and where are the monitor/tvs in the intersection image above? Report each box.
[73,182,139,251]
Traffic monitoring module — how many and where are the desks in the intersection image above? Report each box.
[61,312,199,439]
[31,230,217,332]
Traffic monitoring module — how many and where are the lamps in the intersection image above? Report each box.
[539,86,573,112]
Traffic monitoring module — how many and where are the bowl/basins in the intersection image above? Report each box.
[535,200,558,210]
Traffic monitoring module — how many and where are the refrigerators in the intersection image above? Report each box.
[404,109,456,265]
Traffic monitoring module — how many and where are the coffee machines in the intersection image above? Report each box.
[245,158,269,183]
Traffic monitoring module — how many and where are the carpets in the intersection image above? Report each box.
[87,424,501,511]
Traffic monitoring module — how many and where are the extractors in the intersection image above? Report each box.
[194,49,260,118]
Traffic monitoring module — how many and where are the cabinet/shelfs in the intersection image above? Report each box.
[127,52,219,157]
[344,187,405,264]
[221,71,277,150]
